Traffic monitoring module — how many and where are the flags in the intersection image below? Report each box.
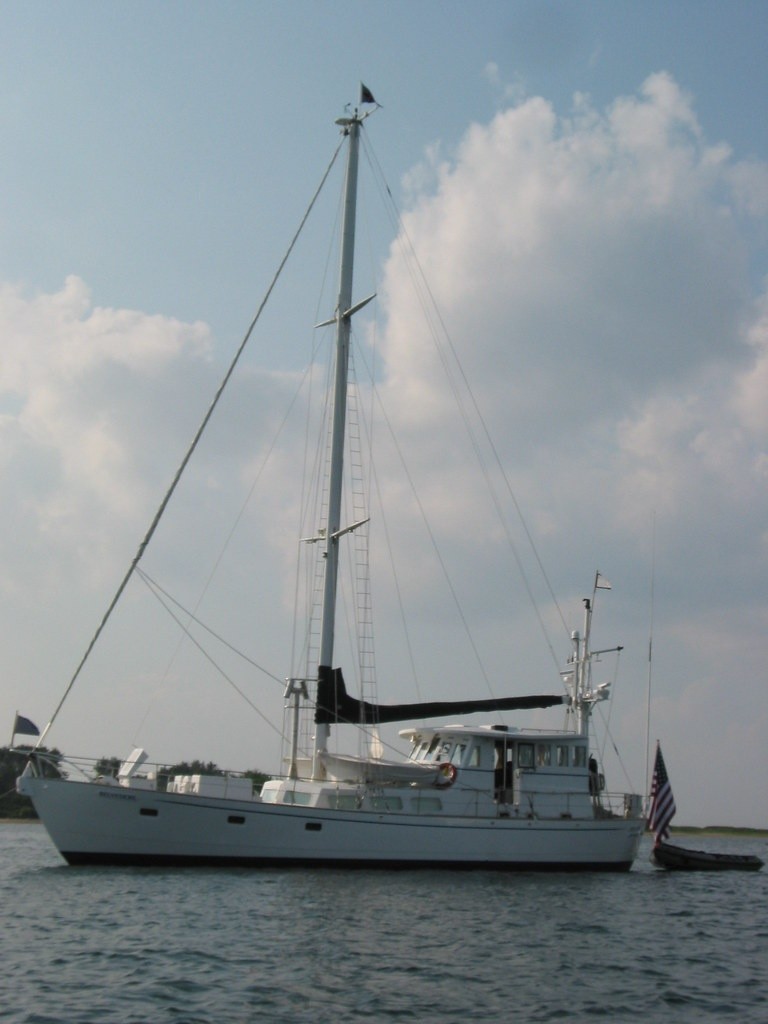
[646,739,677,848]
[361,84,375,102]
[14,715,40,736]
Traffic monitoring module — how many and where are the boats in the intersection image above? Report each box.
[658,833,765,874]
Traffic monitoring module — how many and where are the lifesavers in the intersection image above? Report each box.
[435,763,456,789]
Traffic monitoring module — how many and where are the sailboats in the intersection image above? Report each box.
[15,76,656,872]
